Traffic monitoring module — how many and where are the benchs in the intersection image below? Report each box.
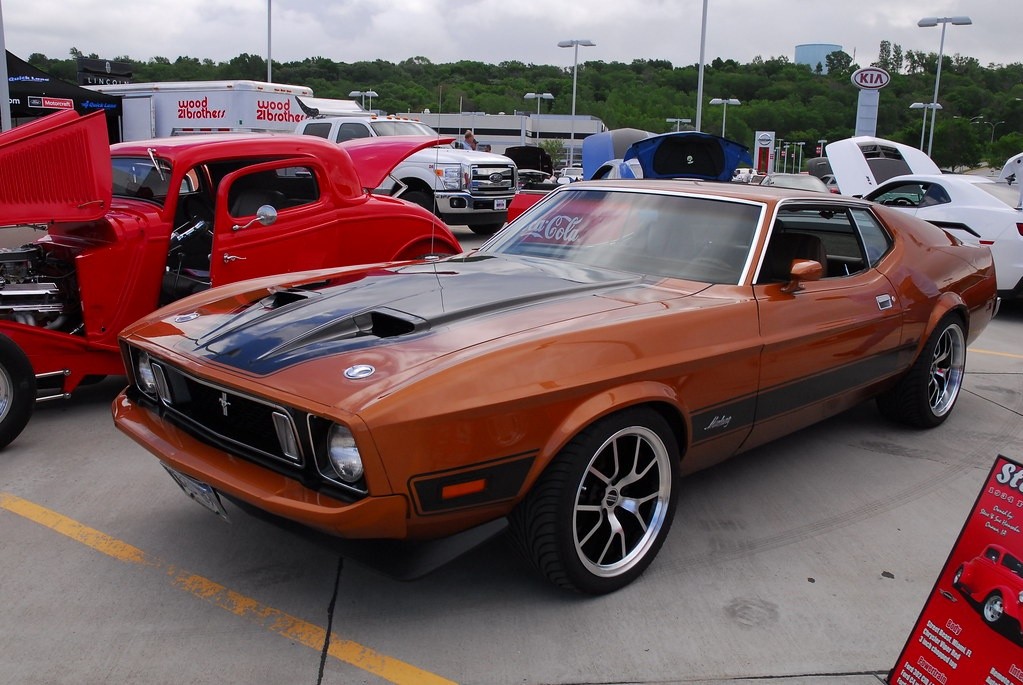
[828,255,864,277]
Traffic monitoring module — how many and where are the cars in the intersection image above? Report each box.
[582,126,755,183]
[116,173,1000,600]
[734,166,833,194]
[503,145,584,188]
[825,134,1023,311]
[0,108,464,453]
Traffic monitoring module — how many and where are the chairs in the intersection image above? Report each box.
[758,235,827,280]
[230,170,285,217]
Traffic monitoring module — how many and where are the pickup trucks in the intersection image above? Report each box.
[505,183,646,246]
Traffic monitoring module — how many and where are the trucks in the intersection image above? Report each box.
[81,81,520,235]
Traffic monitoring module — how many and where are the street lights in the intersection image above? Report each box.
[523,91,554,147]
[775,138,828,175]
[917,17,974,159]
[665,117,692,132]
[984,122,1006,144]
[558,38,597,169]
[348,89,380,109]
[909,102,943,152]
[710,98,742,139]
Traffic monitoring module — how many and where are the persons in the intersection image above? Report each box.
[461,130,479,150]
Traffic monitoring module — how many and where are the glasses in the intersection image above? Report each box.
[484,148,487,149]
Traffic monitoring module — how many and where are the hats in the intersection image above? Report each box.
[473,139,479,144]
